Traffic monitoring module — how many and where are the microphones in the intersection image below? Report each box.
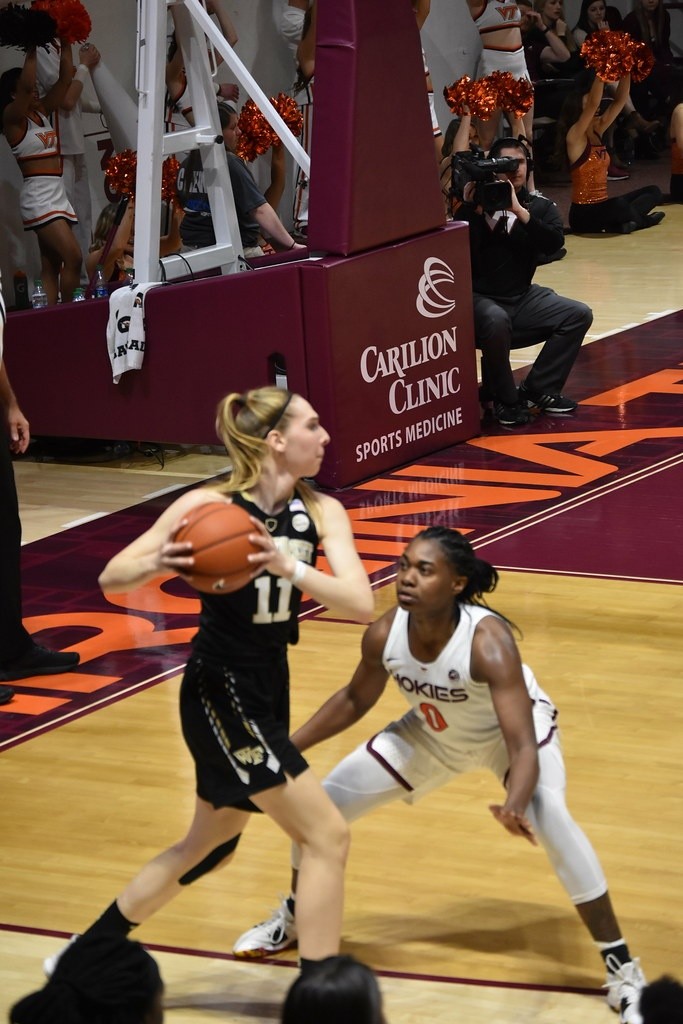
[462,162,488,179]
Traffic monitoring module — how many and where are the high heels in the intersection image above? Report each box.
[625,112,660,138]
[607,147,631,170]
[634,134,663,160]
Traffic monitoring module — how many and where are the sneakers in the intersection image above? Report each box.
[233,892,298,958]
[517,382,578,413]
[600,954,648,1024]
[491,386,528,426]
[43,934,80,980]
[0,637,80,681]
[0,685,14,704]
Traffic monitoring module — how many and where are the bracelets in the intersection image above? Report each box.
[290,562,305,585]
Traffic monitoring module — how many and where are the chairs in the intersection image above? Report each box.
[533,77,669,172]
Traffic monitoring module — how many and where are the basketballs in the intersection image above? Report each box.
[167,500,263,594]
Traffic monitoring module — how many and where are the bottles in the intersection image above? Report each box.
[31,280,48,309]
[13,270,29,306]
[95,265,109,297]
[122,267,134,286]
[72,288,85,302]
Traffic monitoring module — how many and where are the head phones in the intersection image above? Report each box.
[486,133,536,172]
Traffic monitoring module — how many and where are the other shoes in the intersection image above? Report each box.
[606,164,631,181]
[649,212,665,225]
[605,222,637,234]
[537,249,566,265]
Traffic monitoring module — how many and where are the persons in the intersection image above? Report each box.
[0,1,683,703]
[46,387,349,972]
[0,930,682,1024]
[229,528,649,1024]
